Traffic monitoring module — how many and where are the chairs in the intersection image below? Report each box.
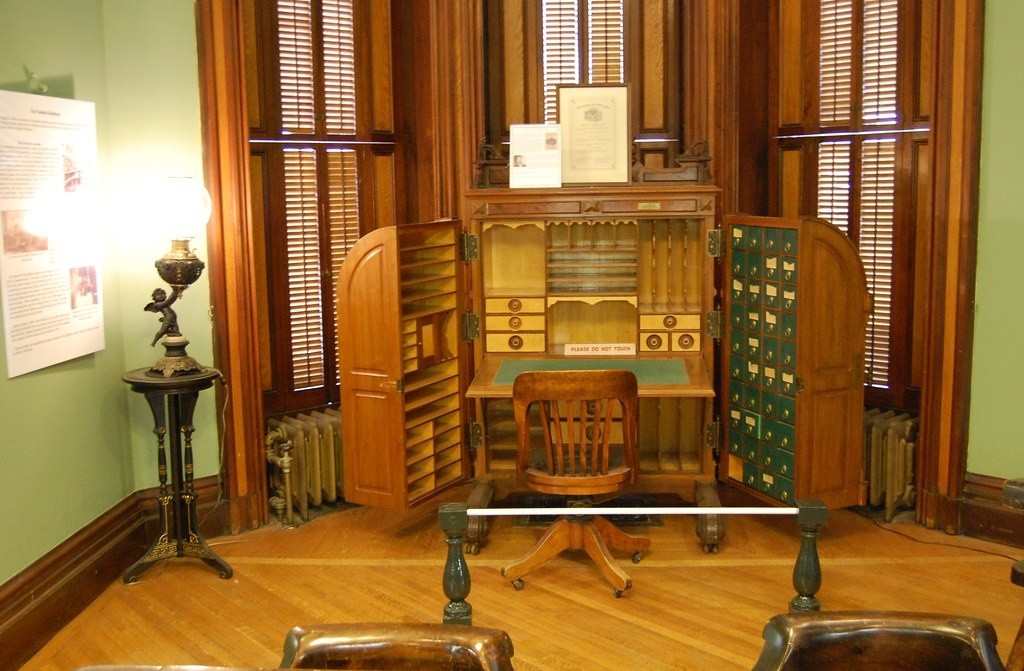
[501,370,651,598]
[278,622,517,671]
[750,610,1007,671]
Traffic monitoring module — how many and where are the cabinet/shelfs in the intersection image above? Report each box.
[337,185,870,553]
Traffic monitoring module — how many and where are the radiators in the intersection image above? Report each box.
[264,407,343,521]
[861,408,920,521]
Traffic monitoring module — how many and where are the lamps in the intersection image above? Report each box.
[138,178,212,377]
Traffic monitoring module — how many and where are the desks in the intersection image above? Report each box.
[123,368,232,584]
